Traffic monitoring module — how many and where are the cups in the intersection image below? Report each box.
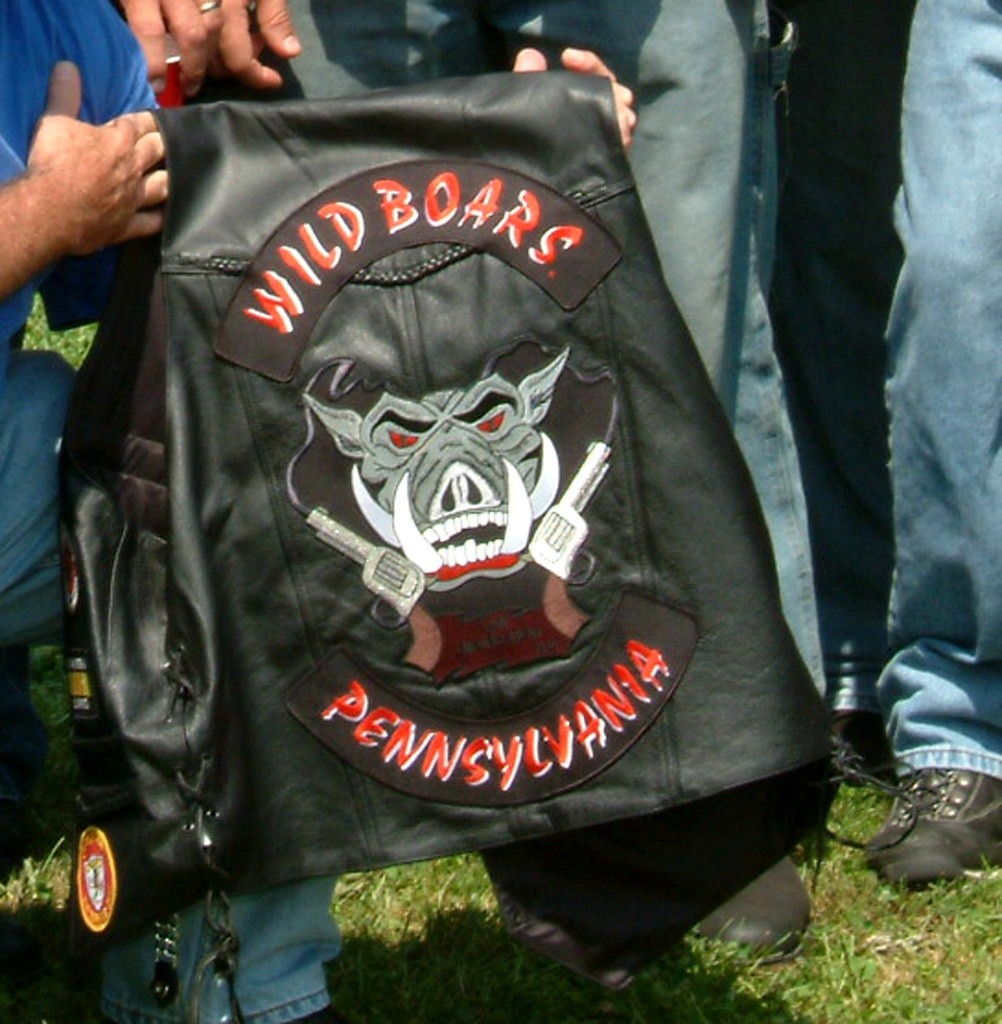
[131,28,187,110]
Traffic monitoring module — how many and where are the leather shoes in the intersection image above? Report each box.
[698,850,813,964]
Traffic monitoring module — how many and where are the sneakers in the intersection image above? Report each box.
[773,706,896,836]
[866,767,1002,890]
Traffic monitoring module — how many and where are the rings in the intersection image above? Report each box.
[202,2,221,11]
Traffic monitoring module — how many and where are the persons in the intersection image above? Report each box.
[0,0,1002,1024]
[0,1,168,986]
